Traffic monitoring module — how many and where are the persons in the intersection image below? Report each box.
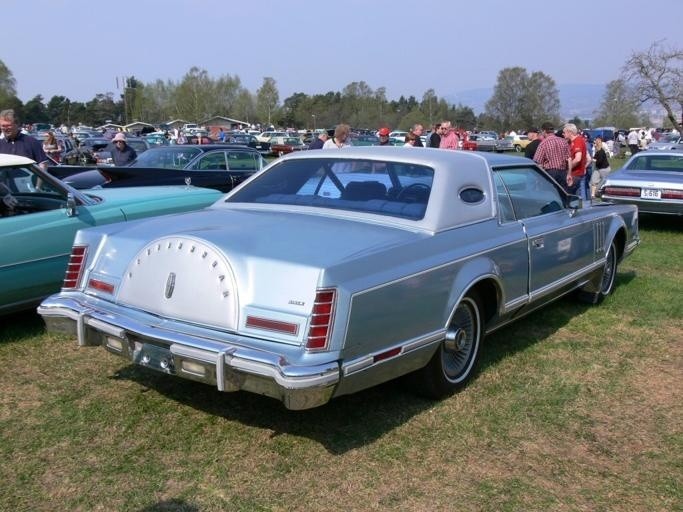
[43,132,62,163]
[111,133,137,166]
[0,109,49,191]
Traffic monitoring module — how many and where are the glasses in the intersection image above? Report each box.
[409,139,417,141]
[440,127,449,130]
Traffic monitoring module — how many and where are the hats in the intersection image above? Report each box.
[523,126,539,136]
[111,133,127,144]
[378,127,390,136]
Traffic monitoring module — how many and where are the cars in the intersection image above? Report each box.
[0,151,227,316]
[26,138,647,420]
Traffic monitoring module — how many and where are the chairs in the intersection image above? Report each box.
[339,181,386,202]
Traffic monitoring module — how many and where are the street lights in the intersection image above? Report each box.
[266,96,271,125]
[311,115,316,130]
